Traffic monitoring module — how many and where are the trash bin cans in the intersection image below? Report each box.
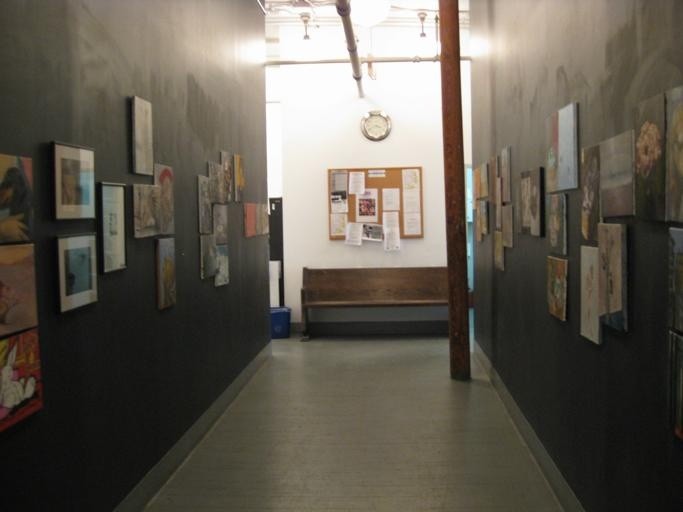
[270,306,291,339]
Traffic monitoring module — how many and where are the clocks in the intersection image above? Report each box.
[360,111,391,141]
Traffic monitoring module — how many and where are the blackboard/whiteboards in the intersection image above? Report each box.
[327,167,424,241]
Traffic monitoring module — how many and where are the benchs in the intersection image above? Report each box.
[300,266,449,337]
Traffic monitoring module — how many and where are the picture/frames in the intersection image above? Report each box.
[99,182,128,273]
[50,140,98,221]
[54,232,100,314]
